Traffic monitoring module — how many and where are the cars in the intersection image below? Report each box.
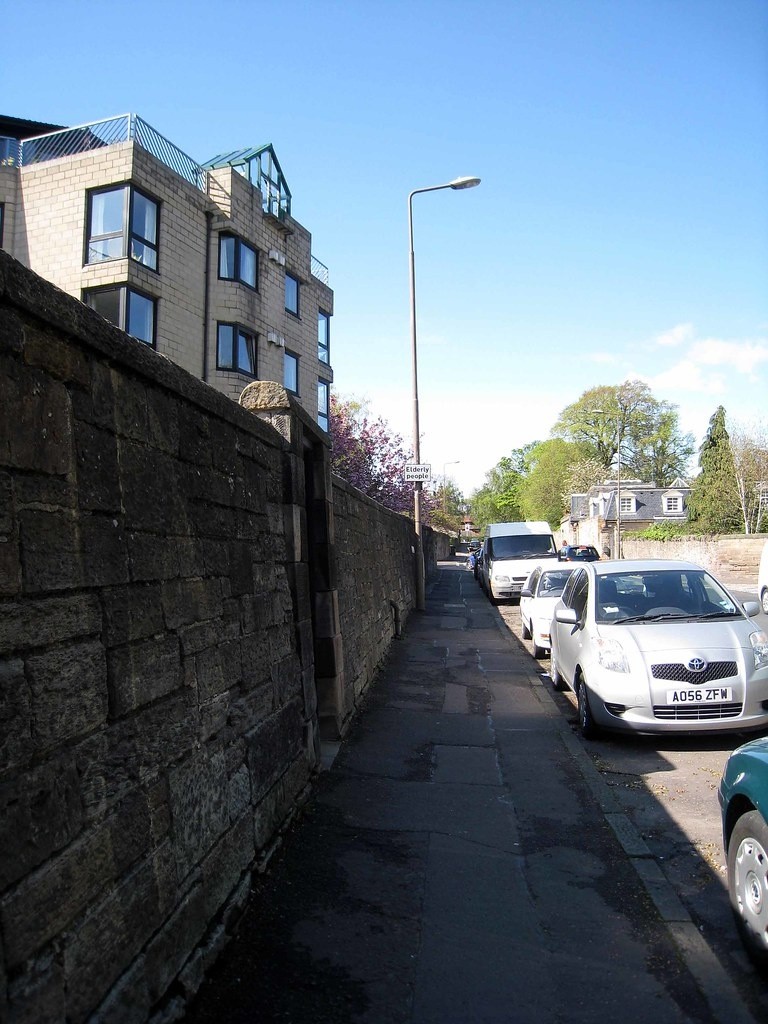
[717,735,768,971]
[758,538,768,615]
[549,558,768,743]
[557,545,602,562]
[466,548,482,580]
[466,540,481,552]
[519,560,635,659]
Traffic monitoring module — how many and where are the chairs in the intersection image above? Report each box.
[587,580,620,614]
[645,581,698,613]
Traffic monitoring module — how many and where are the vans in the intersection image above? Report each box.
[478,521,560,606]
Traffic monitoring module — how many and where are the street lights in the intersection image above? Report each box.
[444,460,460,512]
[592,409,620,560]
[408,176,482,610]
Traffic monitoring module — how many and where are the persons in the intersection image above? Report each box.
[470,553,475,569]
[560,541,572,561]
